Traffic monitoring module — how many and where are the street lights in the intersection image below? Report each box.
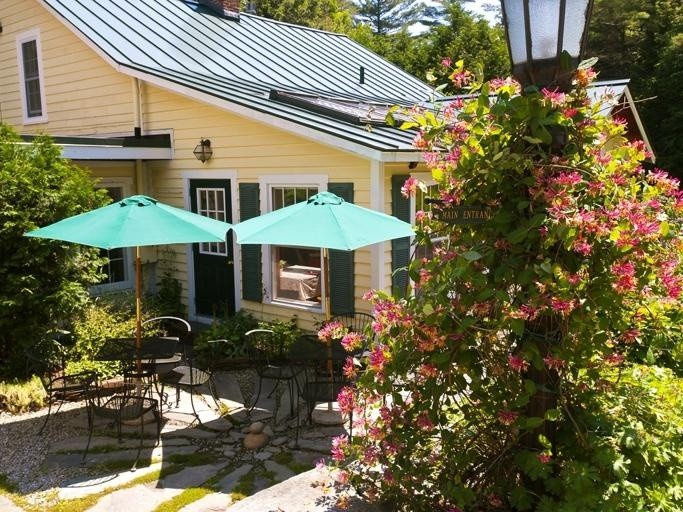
[498,1,590,512]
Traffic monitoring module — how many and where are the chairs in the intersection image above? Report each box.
[133,316,192,401]
[245,328,311,416]
[26,340,96,436]
[80,376,161,472]
[286,311,378,446]
[158,339,231,424]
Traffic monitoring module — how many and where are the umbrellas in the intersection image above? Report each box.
[20,195,235,401]
[229,186,419,412]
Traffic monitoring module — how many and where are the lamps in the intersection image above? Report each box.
[192,139,212,163]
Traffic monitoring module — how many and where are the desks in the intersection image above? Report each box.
[94,337,180,427]
[286,264,321,274]
[280,272,319,301]
